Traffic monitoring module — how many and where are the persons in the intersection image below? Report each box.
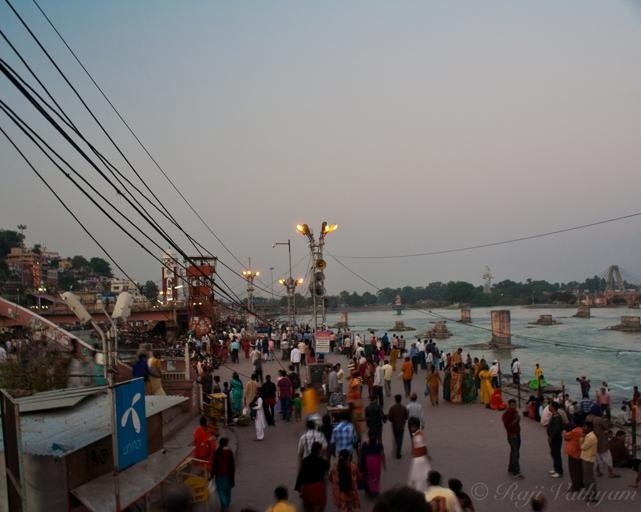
[0,313,641,512]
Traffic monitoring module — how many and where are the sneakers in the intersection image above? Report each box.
[548,470,555,474]
[513,474,525,480]
[551,473,559,478]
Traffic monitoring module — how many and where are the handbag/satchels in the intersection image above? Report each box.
[423,385,428,396]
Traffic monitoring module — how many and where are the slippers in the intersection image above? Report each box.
[597,472,602,477]
[608,474,620,478]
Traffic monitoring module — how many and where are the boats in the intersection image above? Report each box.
[523,379,567,394]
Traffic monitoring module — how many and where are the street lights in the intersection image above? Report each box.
[242,257,259,311]
[16,224,27,253]
[269,266,276,309]
[193,301,204,315]
[278,277,303,338]
[59,290,136,510]
[296,221,337,333]
[36,285,47,311]
[16,287,20,305]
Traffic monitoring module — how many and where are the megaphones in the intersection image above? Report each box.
[310,258,327,299]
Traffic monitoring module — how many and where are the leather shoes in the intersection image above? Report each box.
[568,485,577,492]
